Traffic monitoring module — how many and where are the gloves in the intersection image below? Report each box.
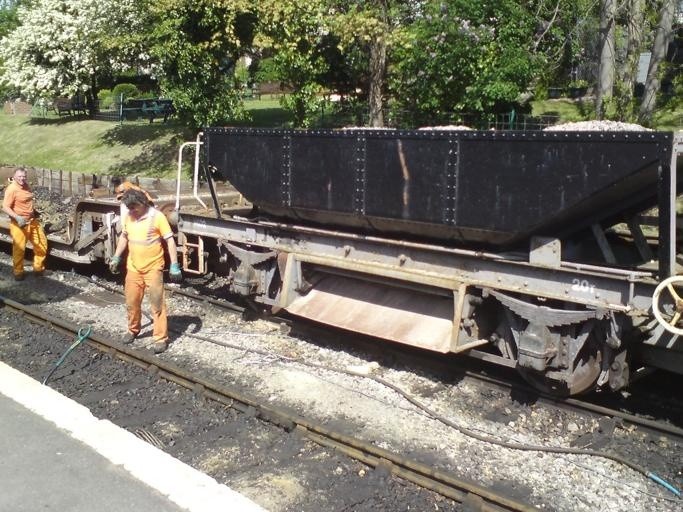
[169,262,182,281]
[109,256,121,274]
[16,217,27,227]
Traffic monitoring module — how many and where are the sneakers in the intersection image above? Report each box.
[33,268,51,277]
[154,341,167,353]
[13,273,25,282]
[123,331,135,344]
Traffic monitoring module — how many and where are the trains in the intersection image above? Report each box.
[0,116,683,401]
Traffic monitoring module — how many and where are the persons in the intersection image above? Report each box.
[107,176,184,355]
[2,168,49,281]
